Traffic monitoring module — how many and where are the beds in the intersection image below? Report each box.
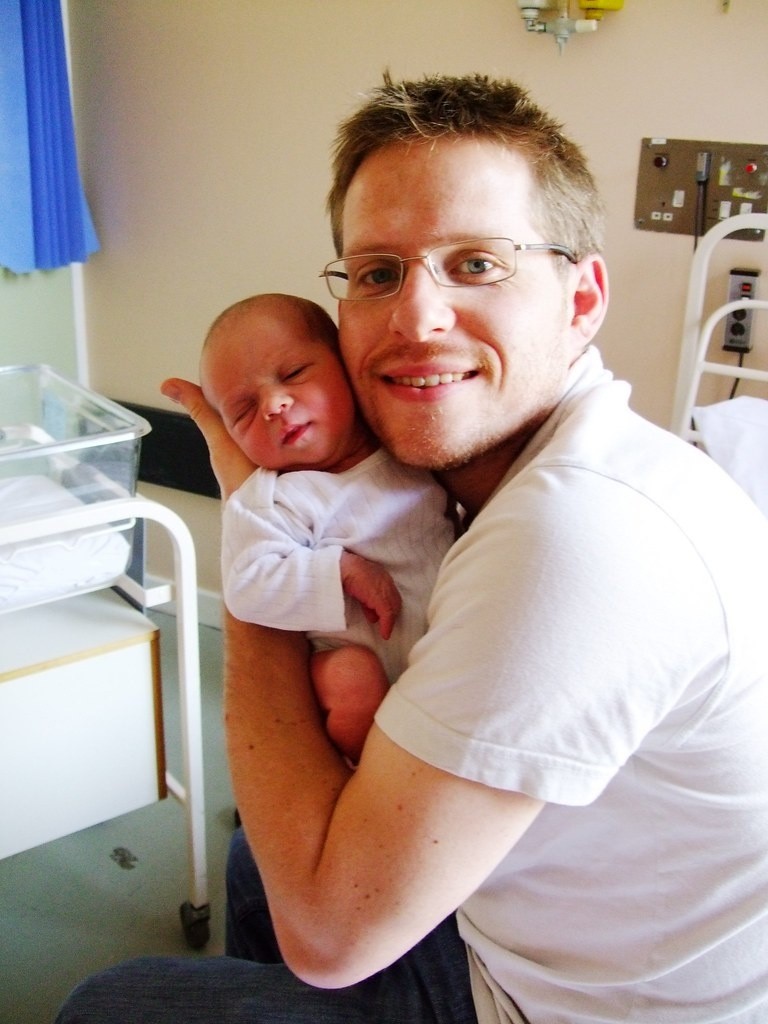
[670,212,768,514]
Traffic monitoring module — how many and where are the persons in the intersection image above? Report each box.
[52,70,768,1024]
[204,294,467,756]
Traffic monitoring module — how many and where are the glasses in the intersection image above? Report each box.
[318,237,578,301]
